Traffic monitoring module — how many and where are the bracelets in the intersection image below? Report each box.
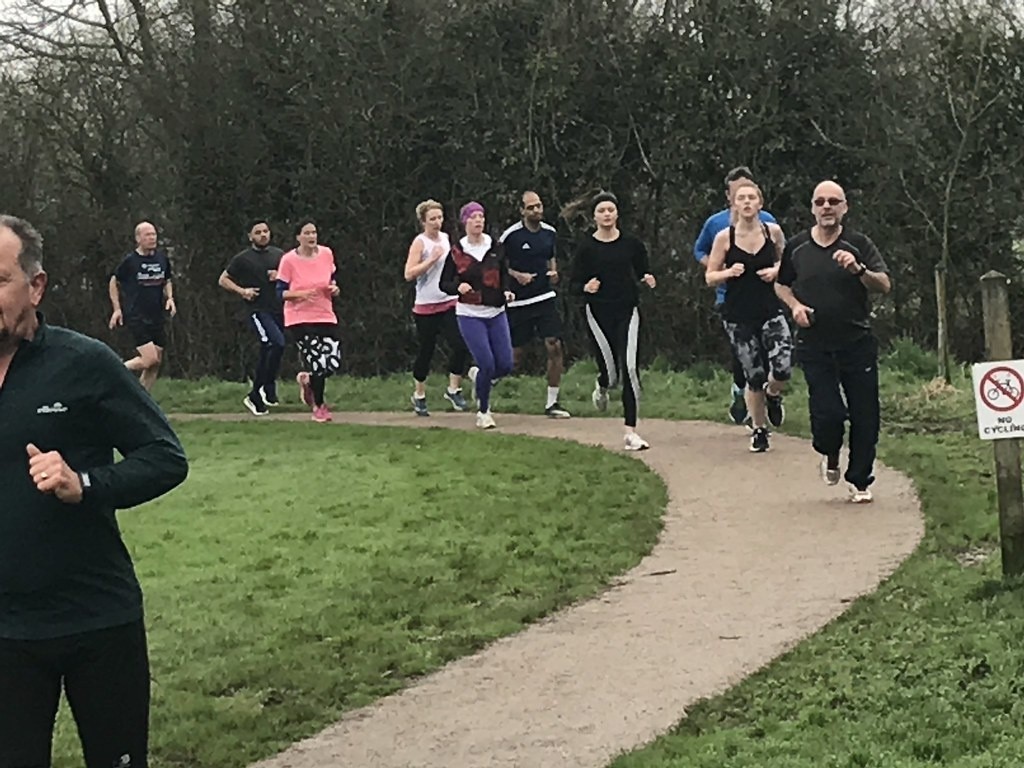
[854,263,866,277]
[168,298,174,301]
[76,471,93,505]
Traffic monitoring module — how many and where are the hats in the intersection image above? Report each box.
[723,165,757,188]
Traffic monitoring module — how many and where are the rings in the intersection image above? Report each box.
[41,472,48,480]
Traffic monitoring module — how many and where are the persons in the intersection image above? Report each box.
[573,192,656,450]
[694,167,776,437]
[704,183,794,453]
[404,199,471,417]
[218,220,287,415]
[0,215,189,768]
[439,202,515,429]
[774,180,891,503]
[108,221,176,392]
[276,219,342,423]
[498,190,571,419]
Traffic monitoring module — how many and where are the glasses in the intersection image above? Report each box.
[526,203,544,210]
[812,197,844,206]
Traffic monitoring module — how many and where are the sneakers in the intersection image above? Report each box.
[309,403,332,423]
[847,483,874,505]
[243,390,269,417]
[625,432,650,451]
[728,382,750,425]
[468,367,479,402]
[748,426,773,453]
[762,381,786,429]
[258,383,280,407]
[746,417,752,431]
[443,387,470,412]
[593,373,609,413]
[296,372,314,406]
[819,456,841,486]
[410,392,430,418]
[475,409,496,430]
[544,402,570,419]
